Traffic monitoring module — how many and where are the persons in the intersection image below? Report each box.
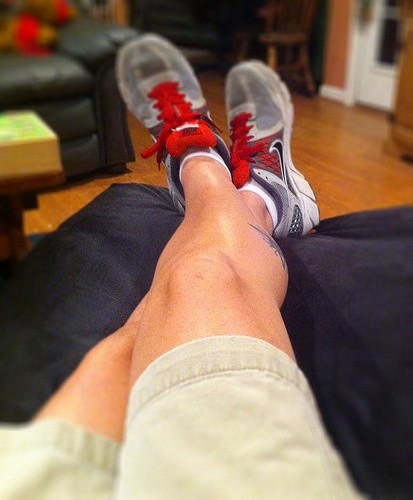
[2,33,365,499]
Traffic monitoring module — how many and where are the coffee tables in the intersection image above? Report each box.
[0,112,65,263]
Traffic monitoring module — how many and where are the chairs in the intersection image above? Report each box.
[253,0,319,94]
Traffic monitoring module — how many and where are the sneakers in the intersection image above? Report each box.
[224,59,322,236]
[115,34,235,216]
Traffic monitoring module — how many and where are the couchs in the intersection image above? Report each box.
[0,0,138,194]
